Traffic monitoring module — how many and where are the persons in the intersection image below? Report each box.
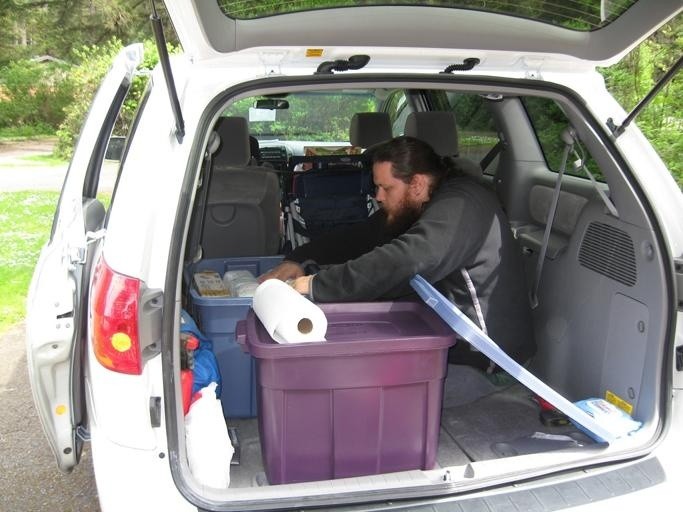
[255,135,539,375]
[249,136,262,161]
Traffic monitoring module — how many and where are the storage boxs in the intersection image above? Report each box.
[233,302,458,487]
[181,254,286,419]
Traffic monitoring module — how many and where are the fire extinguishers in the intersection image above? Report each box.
[179,332,199,414]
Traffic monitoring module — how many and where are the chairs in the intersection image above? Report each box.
[404,108,484,179]
[349,112,393,149]
[187,116,282,262]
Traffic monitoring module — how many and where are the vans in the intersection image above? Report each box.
[20,0,683,512]
